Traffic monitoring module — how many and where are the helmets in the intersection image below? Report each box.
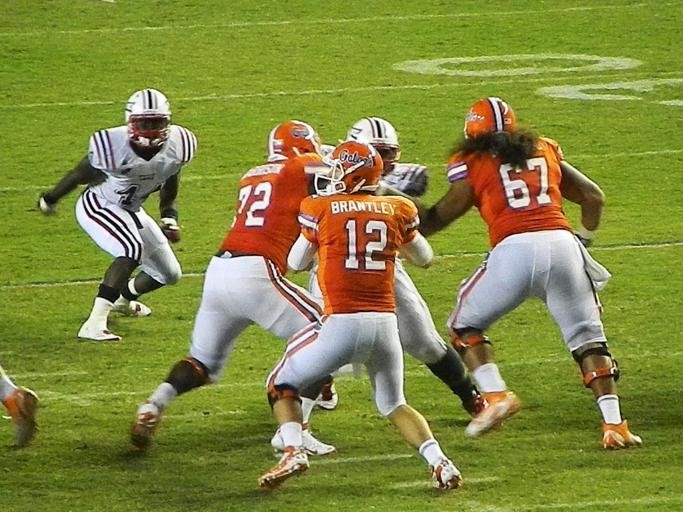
[463,95,518,141]
[313,140,385,197]
[123,87,172,150]
[267,118,322,161]
[346,115,402,163]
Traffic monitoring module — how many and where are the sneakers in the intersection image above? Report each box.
[78,321,121,341]
[315,380,338,410]
[4,385,39,447]
[129,398,163,450]
[269,423,336,457]
[111,295,152,317]
[465,389,522,437]
[428,458,463,490]
[256,445,310,488]
[600,418,643,450]
[462,391,505,430]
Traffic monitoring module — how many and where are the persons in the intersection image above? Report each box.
[317,117,488,419]
[424,96,644,449]
[0,362,39,448]
[257,141,463,493]
[39,88,192,342]
[130,118,339,454]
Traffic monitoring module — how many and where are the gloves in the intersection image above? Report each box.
[159,208,181,243]
[36,191,54,217]
